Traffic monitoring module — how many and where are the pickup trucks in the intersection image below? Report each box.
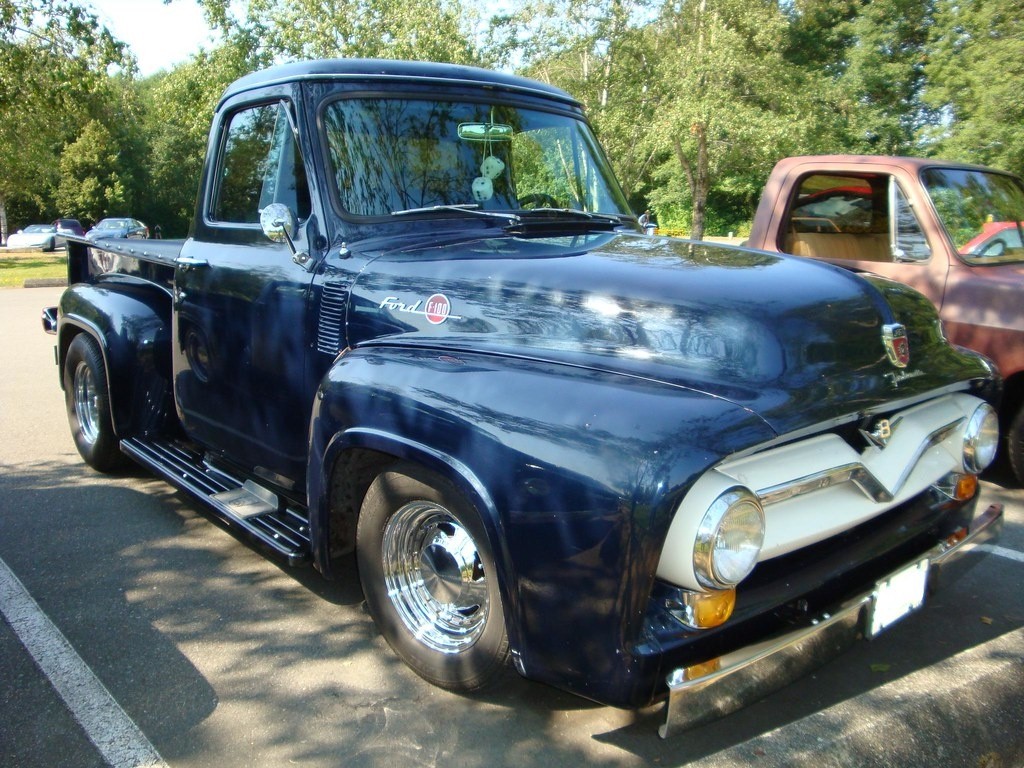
[39,57,1004,736]
[673,153,1024,488]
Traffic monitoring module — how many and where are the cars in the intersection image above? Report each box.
[6,223,67,252]
[49,218,85,237]
[84,217,149,240]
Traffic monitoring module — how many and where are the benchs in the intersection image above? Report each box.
[784,230,891,259]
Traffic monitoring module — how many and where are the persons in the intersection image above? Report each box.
[154,225,162,239]
[87,222,94,232]
